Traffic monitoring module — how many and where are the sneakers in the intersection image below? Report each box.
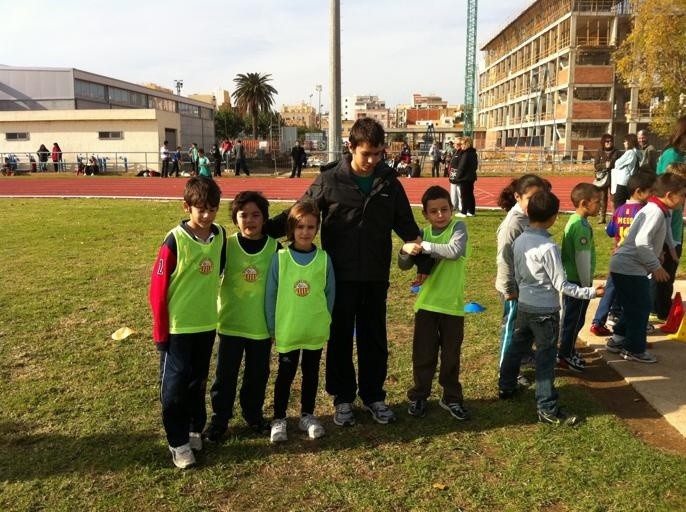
[590,322,610,336]
[407,400,426,419]
[620,341,657,363]
[334,403,355,427]
[201,425,227,444]
[521,349,538,364]
[498,388,525,400]
[271,418,288,442]
[364,401,396,425]
[299,413,326,439]
[439,397,468,420]
[517,372,530,387]
[455,212,466,218]
[606,334,626,353]
[556,351,585,375]
[188,432,203,452]
[246,418,271,435]
[466,213,475,216]
[646,322,655,334]
[169,442,196,469]
[537,409,577,428]
[607,315,619,326]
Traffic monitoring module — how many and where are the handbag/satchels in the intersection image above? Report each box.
[449,168,458,184]
[592,170,610,189]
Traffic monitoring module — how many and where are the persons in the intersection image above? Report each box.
[609,172,686,365]
[653,116,686,327]
[447,137,464,212]
[262,201,337,444]
[288,140,304,178]
[556,183,603,370]
[160,140,170,177]
[439,152,452,177]
[648,161,685,317]
[493,173,553,400]
[589,166,658,337]
[188,143,200,177]
[445,141,457,155]
[200,190,282,450]
[412,158,421,177]
[210,144,222,177]
[37,144,50,171]
[429,140,442,177]
[170,147,183,177]
[400,143,412,164]
[509,193,606,426]
[232,140,250,177]
[342,140,350,154]
[149,174,228,469]
[609,134,641,196]
[52,143,62,172]
[261,116,434,426]
[396,160,411,178]
[397,184,471,421]
[637,129,657,188]
[590,133,621,225]
[197,149,212,180]
[608,184,633,323]
[455,137,478,219]
[220,136,233,168]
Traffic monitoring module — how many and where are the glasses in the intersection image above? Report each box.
[605,140,613,142]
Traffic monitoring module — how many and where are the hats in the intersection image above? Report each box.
[449,141,454,144]
[434,140,438,144]
